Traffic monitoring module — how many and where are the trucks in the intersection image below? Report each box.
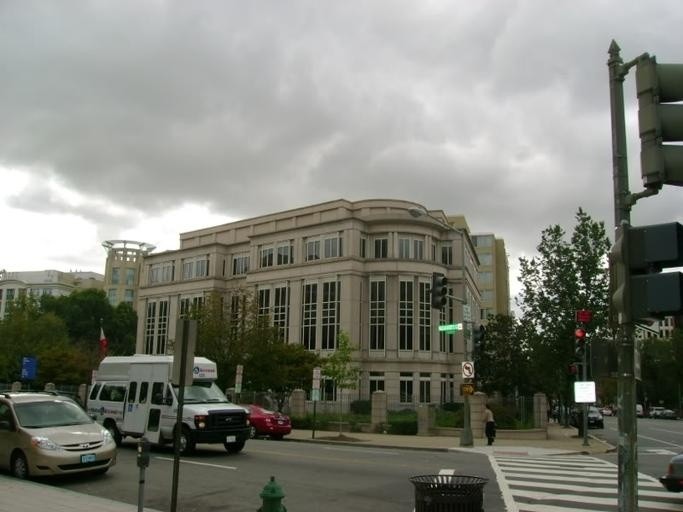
[88,352,251,455]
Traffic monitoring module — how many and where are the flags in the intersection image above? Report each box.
[97,327,107,353]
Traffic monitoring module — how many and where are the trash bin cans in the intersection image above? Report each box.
[408,474,489,512]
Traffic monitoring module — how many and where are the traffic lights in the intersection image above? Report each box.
[574,328,584,356]
[620,219,680,320]
[434,275,447,306]
[636,56,682,190]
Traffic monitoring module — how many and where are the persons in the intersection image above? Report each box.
[482,403,497,446]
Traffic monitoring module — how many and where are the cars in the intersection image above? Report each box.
[659,452,683,491]
[0,391,119,479]
[550,403,676,427]
[238,403,293,441]
